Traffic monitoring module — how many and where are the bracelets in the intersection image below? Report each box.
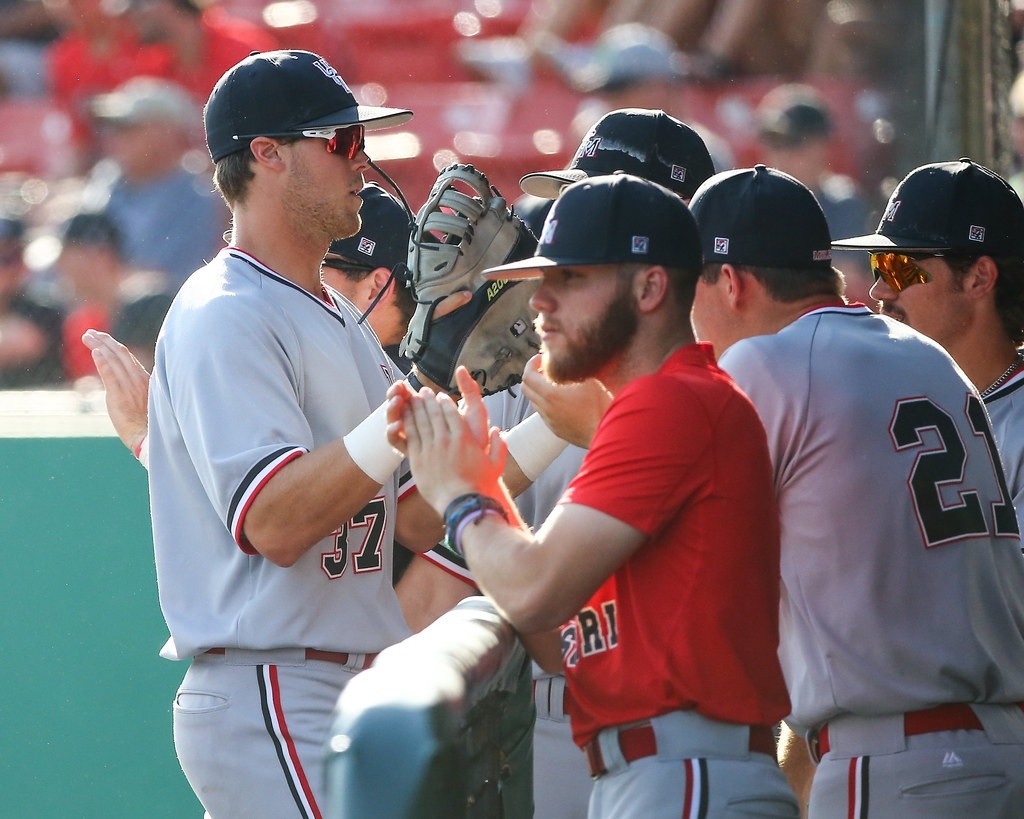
[442,493,480,519]
[455,509,508,556]
[447,497,510,556]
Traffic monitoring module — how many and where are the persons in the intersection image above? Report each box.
[0,0,1024,819]
[148,46,568,819]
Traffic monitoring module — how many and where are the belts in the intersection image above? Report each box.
[806,701,1024,773]
[204,648,379,670]
[584,724,777,781]
[532,679,574,715]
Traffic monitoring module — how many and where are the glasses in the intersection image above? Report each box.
[868,252,957,294]
[233,123,364,160]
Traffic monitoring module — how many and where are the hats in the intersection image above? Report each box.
[519,107,715,199]
[750,82,837,150]
[327,182,414,281]
[687,164,831,270]
[831,158,1024,259]
[480,169,701,279]
[506,192,557,241]
[86,75,205,132]
[573,24,687,96]
[204,49,414,165]
